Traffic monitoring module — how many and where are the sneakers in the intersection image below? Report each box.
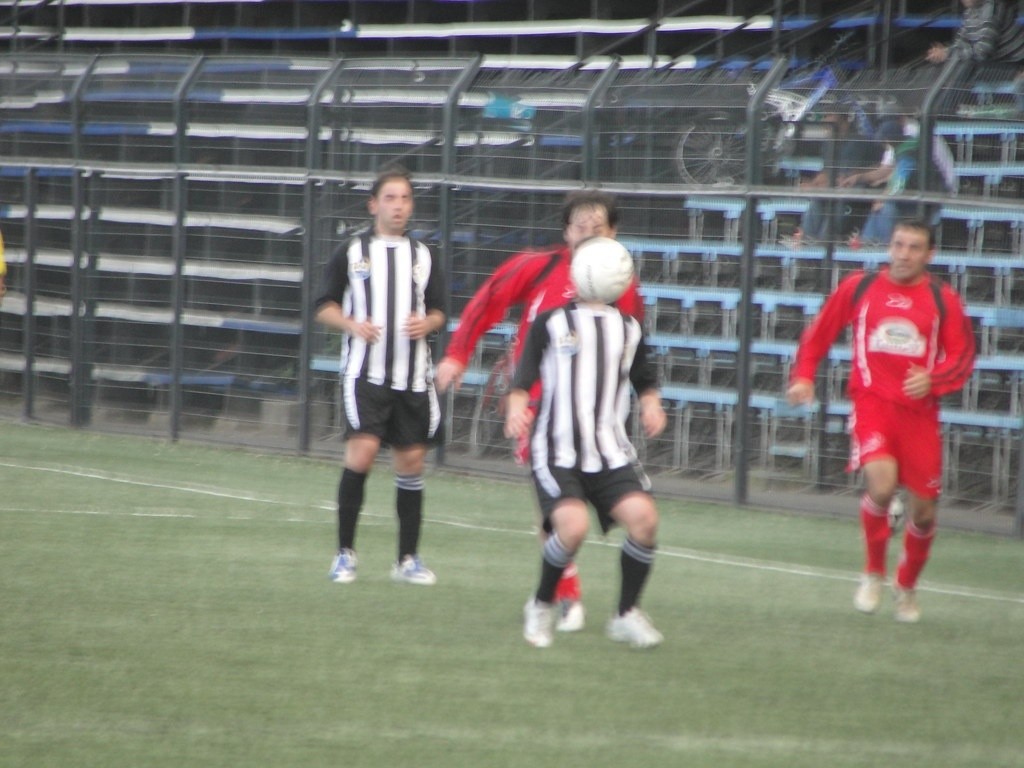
[521,597,554,648]
[603,605,663,649]
[390,557,436,586]
[327,552,358,584]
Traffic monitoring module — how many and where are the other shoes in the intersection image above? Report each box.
[854,571,885,613]
[554,599,586,631]
[894,580,919,624]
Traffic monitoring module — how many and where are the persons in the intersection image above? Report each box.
[801,94,955,251]
[436,194,671,648]
[789,219,975,622]
[309,170,451,586]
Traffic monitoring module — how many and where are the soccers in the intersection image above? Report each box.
[568,237,635,305]
